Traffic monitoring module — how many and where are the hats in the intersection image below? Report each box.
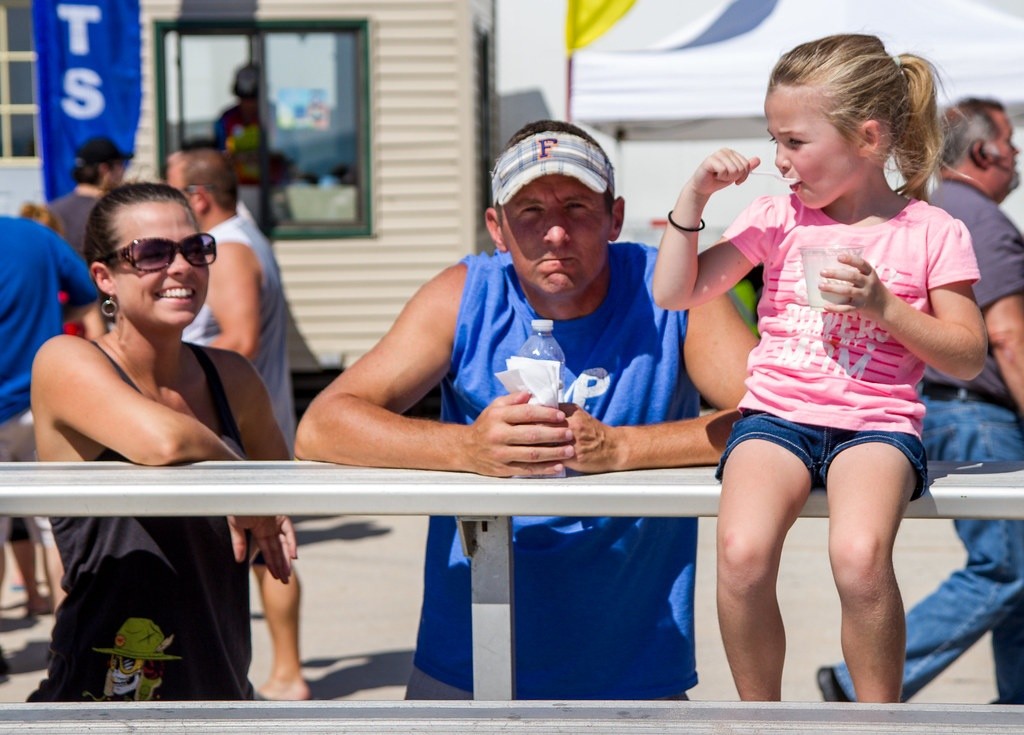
[491,132,617,206]
[80,137,134,165]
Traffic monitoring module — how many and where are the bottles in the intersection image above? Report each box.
[514,319,567,479]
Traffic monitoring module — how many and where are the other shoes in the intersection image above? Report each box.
[817,667,845,702]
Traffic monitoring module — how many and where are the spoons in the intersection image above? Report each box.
[749,171,801,186]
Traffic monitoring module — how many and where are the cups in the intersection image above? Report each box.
[797,243,865,312]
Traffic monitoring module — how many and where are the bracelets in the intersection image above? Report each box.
[668,210,706,233]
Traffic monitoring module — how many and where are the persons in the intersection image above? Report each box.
[0,138,310,702]
[210,67,277,186]
[816,98,1024,704]
[293,119,760,700]
[653,35,988,704]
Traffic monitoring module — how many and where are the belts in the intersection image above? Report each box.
[923,379,1020,416]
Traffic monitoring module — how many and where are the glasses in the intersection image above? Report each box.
[96,232,216,273]
